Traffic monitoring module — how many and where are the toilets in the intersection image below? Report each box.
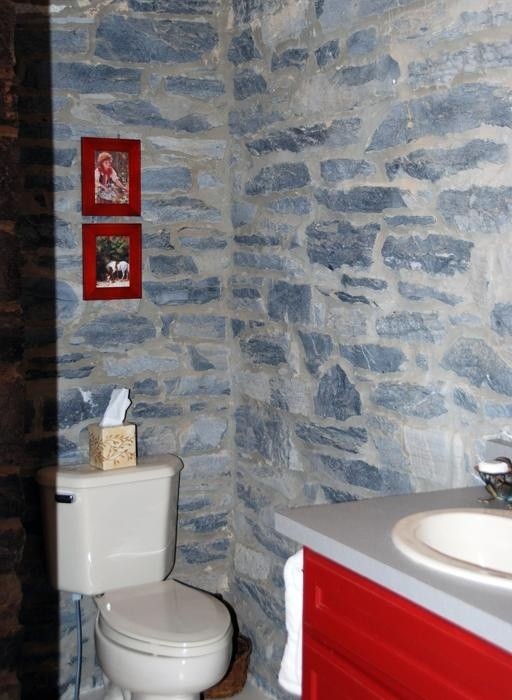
[37,453,234,700]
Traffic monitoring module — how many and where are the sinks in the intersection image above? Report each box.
[391,507,512,585]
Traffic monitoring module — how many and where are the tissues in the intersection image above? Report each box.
[88,388,137,472]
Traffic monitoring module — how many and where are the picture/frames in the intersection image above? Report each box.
[82,135,143,302]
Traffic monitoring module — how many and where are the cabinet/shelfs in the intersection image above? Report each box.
[301,546,511,700]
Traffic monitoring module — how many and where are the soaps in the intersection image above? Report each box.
[478,460,507,474]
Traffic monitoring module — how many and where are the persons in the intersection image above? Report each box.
[105,260,116,283]
[116,260,129,282]
[93,151,129,204]
